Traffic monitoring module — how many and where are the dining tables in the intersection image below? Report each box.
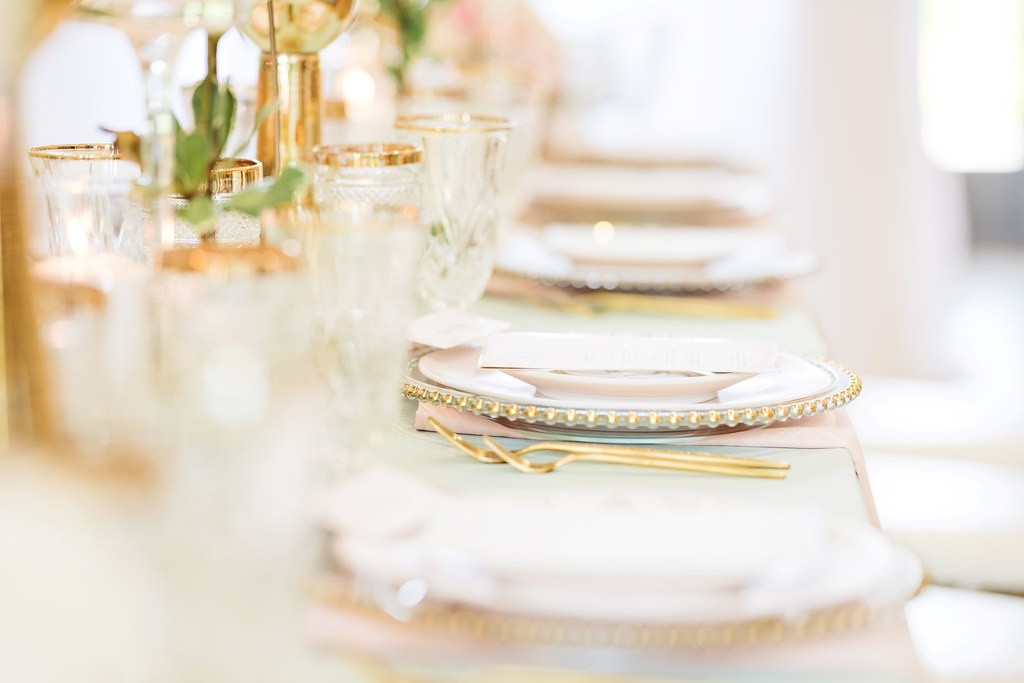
[0,117,923,683]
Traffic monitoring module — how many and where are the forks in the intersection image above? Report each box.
[423,414,791,470]
[483,433,786,478]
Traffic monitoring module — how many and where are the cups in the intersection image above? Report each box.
[28,115,512,499]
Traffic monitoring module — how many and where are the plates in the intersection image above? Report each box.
[499,367,757,405]
[492,227,816,290]
[402,340,864,436]
